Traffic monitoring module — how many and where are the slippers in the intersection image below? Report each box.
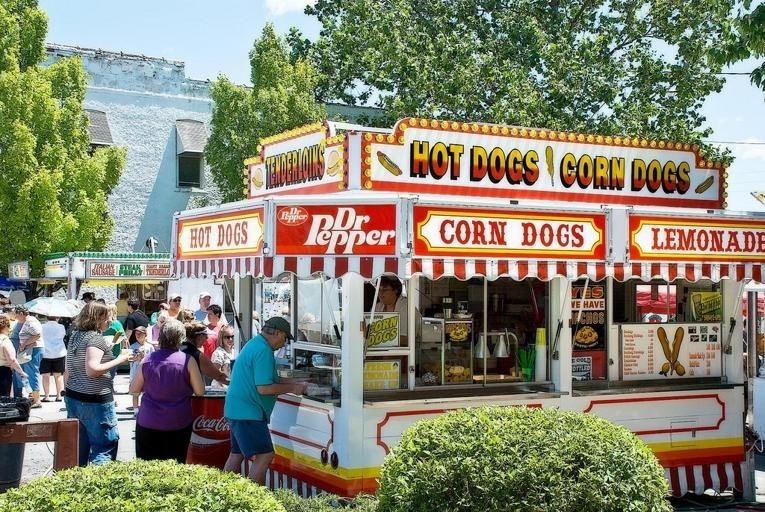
[40,398,50,402]
[55,398,62,402]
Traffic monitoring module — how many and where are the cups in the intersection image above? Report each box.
[534,328,547,383]
[521,368,532,384]
[442,297,454,318]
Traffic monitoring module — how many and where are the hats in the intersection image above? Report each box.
[199,292,211,299]
[133,326,147,334]
[81,292,95,300]
[168,293,183,304]
[264,317,296,342]
[195,327,216,335]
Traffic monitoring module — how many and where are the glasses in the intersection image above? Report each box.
[223,335,234,339]
[170,299,181,302]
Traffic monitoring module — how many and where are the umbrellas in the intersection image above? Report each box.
[19,295,81,318]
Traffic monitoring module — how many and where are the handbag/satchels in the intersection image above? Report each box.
[17,344,33,364]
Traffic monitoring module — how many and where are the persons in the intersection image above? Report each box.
[0,313,28,396]
[128,318,206,463]
[0,275,238,412]
[221,316,306,487]
[126,324,155,409]
[372,274,420,347]
[39,316,67,403]
[7,303,43,409]
[62,300,136,466]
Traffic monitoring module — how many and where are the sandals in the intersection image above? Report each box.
[30,403,42,408]
